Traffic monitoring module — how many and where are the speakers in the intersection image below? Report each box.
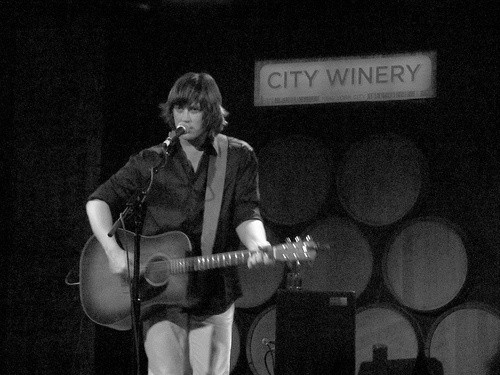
[274,289,356,375]
[359,358,444,375]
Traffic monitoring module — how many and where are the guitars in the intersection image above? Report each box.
[79,228,330,331]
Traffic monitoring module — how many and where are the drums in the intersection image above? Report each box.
[337,133,428,227]
[424,300,500,375]
[249,133,337,226]
[235,225,287,307]
[382,214,473,314]
[230,309,244,375]
[355,303,424,375]
[244,304,276,375]
[289,218,376,301]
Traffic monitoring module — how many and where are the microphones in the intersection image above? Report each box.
[162,122,190,148]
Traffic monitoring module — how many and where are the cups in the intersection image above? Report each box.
[285,272,303,289]
[373,343,387,362]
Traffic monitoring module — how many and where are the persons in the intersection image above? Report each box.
[86,72,275,375]
